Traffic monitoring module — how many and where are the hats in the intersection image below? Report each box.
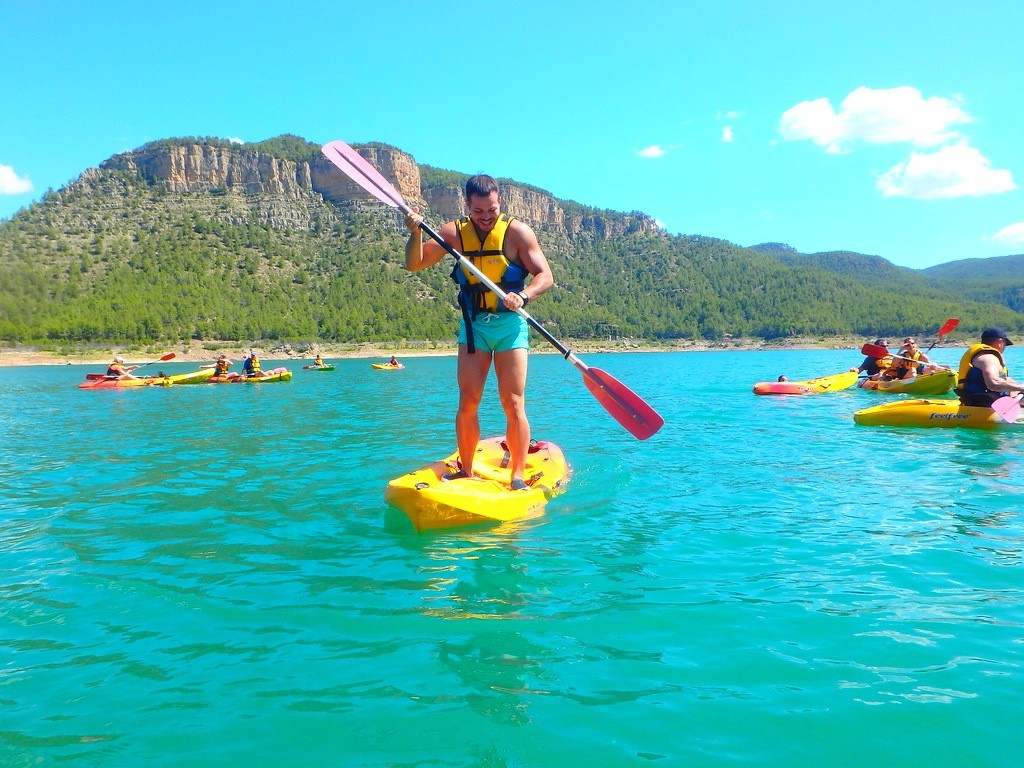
[251,352,257,355]
[981,327,1014,345]
[115,356,124,361]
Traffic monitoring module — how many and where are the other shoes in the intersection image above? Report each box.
[442,471,469,481]
[511,479,529,491]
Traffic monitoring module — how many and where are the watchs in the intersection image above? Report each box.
[518,291,528,307]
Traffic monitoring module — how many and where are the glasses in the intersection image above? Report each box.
[880,345,889,347]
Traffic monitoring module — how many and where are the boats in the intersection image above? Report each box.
[371,362,406,370]
[318,365,336,372]
[79,369,217,388]
[206,367,293,382]
[858,369,959,394]
[383,432,566,533]
[854,399,1024,426]
[751,371,858,394]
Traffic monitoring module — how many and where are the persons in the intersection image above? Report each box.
[199,354,238,378]
[953,327,1024,407]
[385,356,398,366]
[241,352,273,377]
[778,375,788,382]
[404,174,554,490]
[314,355,328,368]
[107,358,138,377]
[850,337,938,382]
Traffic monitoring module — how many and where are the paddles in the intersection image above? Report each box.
[991,389,1024,424]
[225,367,287,380]
[925,318,959,354]
[86,373,161,380]
[858,375,874,379]
[302,363,339,369]
[128,353,176,373]
[861,343,960,374]
[321,140,665,441]
